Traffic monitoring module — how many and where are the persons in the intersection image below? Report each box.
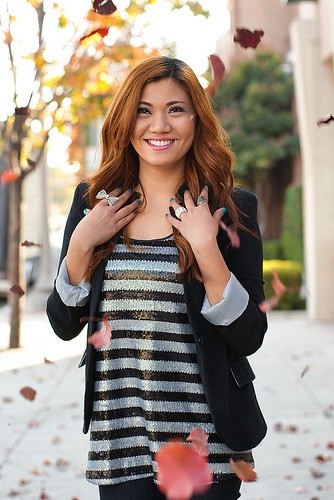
[46,56,269,500]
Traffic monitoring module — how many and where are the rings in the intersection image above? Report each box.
[175,206,187,219]
[196,196,208,205]
[95,189,119,207]
